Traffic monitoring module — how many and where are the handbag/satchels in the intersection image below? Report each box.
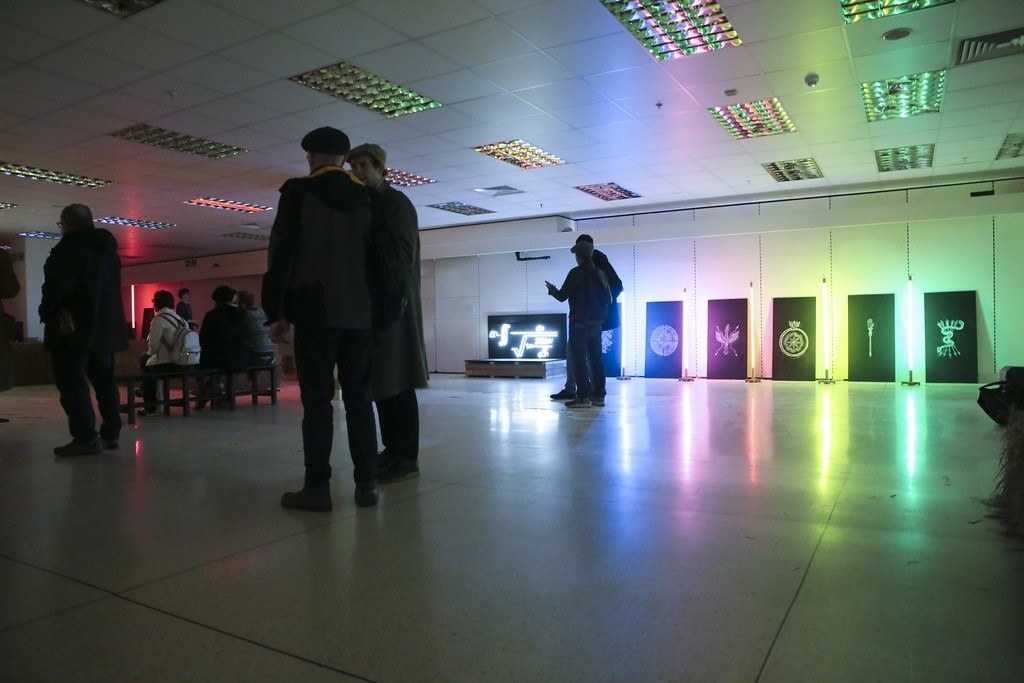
[602,298,619,330]
[368,276,407,331]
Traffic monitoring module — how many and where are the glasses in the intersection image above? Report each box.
[57,221,64,227]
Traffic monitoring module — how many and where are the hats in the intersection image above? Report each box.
[346,143,386,166]
[570,241,593,257]
[211,286,237,302]
[302,126,349,154]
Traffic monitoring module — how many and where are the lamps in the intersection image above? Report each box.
[616,287,631,381]
[678,288,694,382]
[819,279,835,385]
[745,281,762,384]
[901,275,922,386]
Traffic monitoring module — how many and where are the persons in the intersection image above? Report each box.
[135,290,190,417]
[194,286,273,411]
[545,234,623,407]
[261,127,405,511]
[345,144,429,486]
[38,203,130,456]
[177,289,194,330]
[0,249,20,423]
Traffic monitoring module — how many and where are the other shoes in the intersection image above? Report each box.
[355,486,378,505]
[281,486,331,511]
[137,408,156,416]
[54,438,102,456]
[100,437,119,448]
[194,400,207,409]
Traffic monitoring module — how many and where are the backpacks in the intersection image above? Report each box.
[156,313,201,365]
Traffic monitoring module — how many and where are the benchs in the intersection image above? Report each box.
[114,363,278,426]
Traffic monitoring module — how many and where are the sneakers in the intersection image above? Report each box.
[550,390,576,399]
[379,447,419,484]
[591,400,605,405]
[565,400,590,408]
